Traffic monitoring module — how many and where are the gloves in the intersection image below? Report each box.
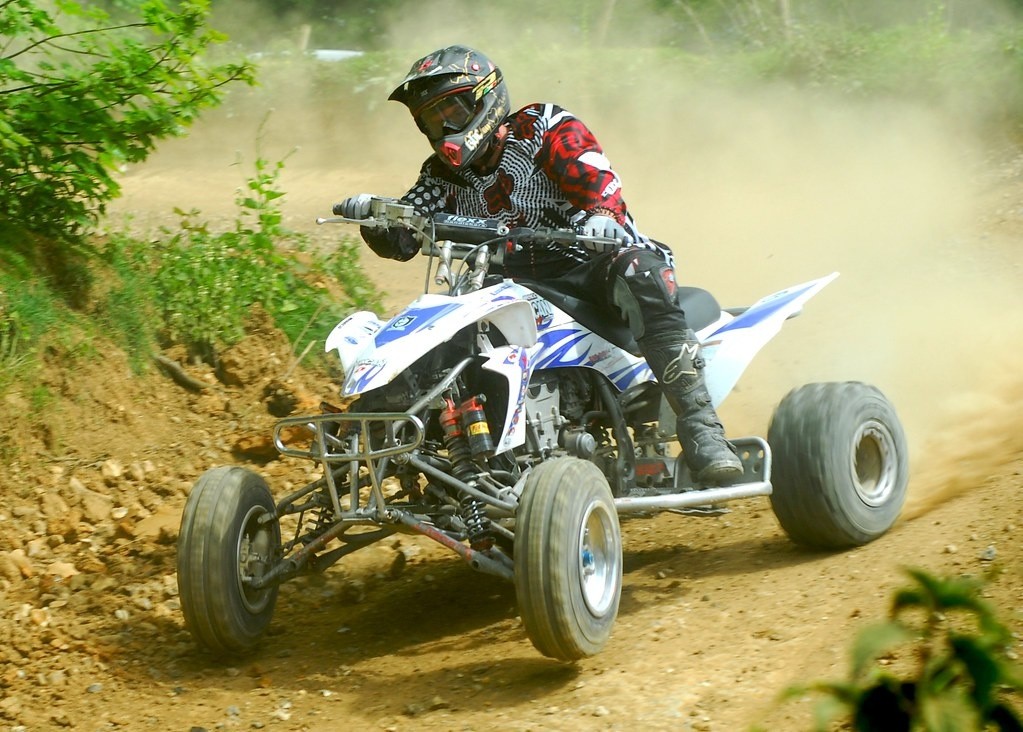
[582,213,632,255]
[341,194,378,220]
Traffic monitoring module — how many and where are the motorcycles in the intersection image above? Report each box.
[177,193,908,660]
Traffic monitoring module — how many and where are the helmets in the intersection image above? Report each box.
[388,44,510,172]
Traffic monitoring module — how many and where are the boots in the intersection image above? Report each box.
[638,328,744,485]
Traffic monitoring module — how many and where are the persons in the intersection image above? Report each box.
[339,43,747,489]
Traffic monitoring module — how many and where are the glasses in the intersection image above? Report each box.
[416,90,477,140]
